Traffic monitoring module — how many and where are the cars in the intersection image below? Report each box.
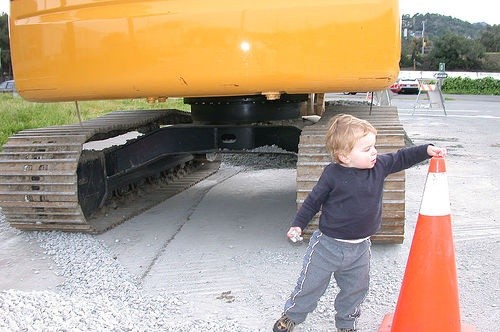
[398,78,420,95]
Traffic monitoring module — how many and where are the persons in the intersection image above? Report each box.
[273,115,447,332]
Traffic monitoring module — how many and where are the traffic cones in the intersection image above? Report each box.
[377,154,476,332]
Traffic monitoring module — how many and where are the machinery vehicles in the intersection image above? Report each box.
[0,0,406,245]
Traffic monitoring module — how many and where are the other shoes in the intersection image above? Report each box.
[338,329,355,332]
[272,315,298,331]
